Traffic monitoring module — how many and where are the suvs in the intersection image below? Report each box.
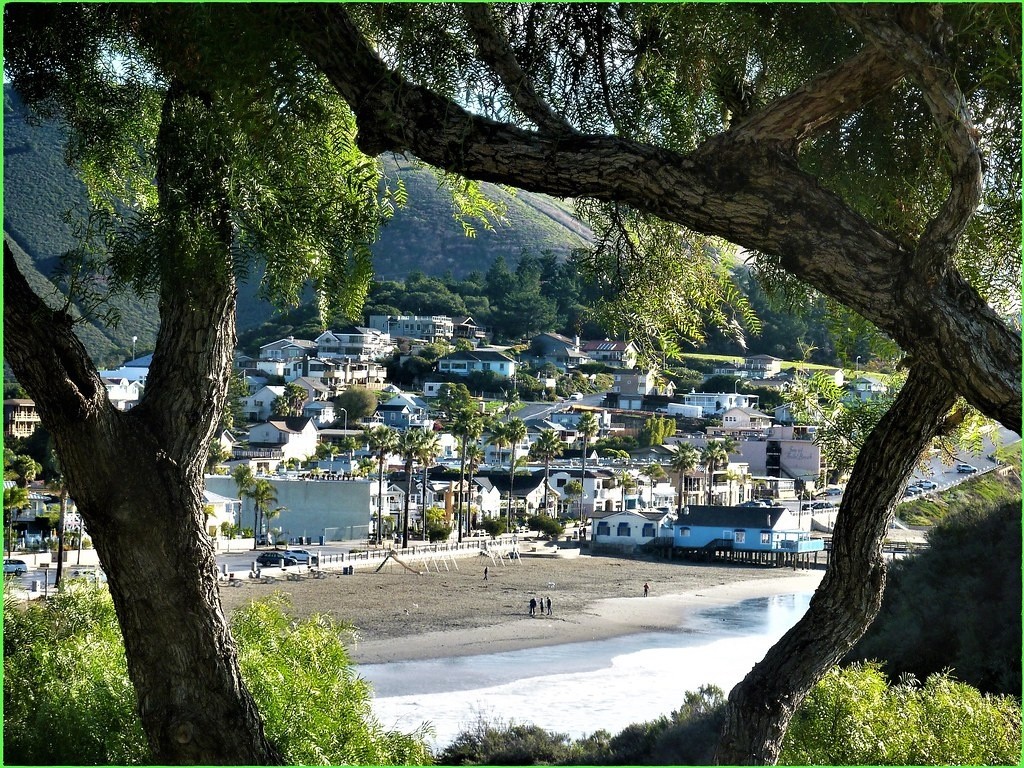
[957,464,977,473]
[3,559,27,576]
[256,551,297,567]
[284,549,317,564]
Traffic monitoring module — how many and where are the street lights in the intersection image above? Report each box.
[856,356,861,380]
[341,407,347,443]
[735,379,741,394]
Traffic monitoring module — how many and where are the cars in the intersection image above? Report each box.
[257,534,272,545]
[736,498,774,508]
[797,488,843,500]
[904,479,938,496]
[70,569,108,584]
[802,502,832,510]
[569,392,583,400]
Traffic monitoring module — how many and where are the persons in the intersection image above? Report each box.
[583,526,586,537]
[546,597,552,615]
[530,598,536,614]
[540,598,544,615]
[644,583,649,597]
[483,567,488,580]
